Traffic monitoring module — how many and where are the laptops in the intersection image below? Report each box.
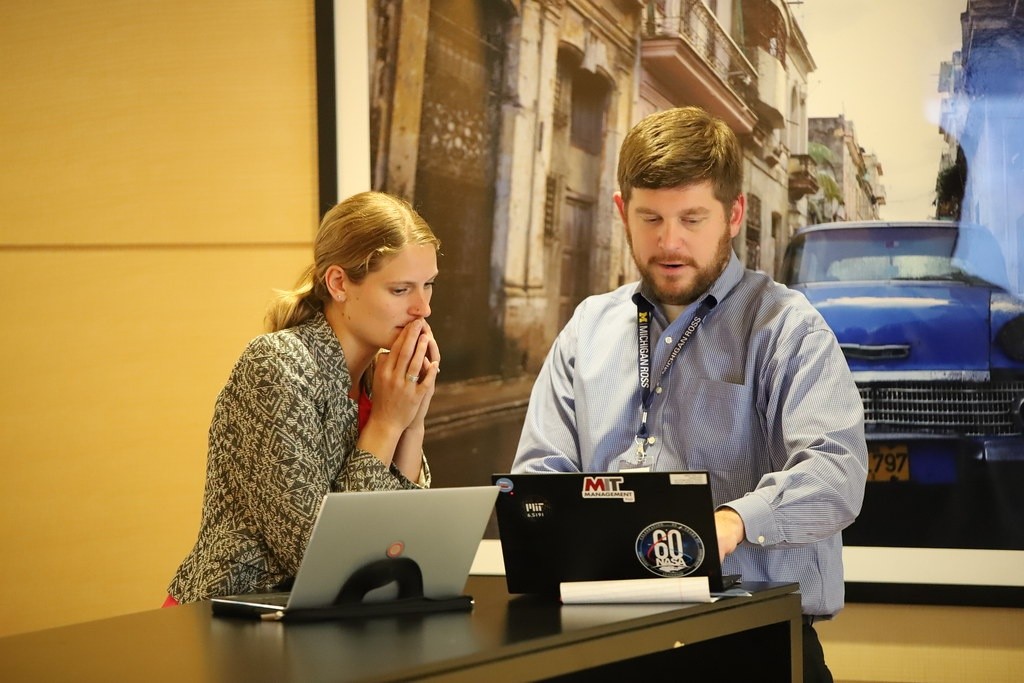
[210,486,501,623]
[490,469,743,593]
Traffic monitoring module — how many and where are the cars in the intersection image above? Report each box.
[775,215,1024,518]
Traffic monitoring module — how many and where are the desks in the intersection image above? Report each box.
[1,573,806,683]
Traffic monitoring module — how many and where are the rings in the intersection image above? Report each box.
[404,373,420,382]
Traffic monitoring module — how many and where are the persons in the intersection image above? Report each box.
[158,191,441,611]
[510,108,867,683]
